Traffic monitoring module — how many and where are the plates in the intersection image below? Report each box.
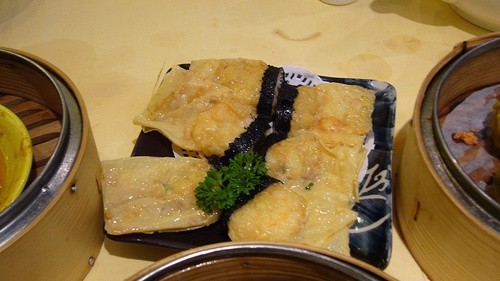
[104,62,397,272]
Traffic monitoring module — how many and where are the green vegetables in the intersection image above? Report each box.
[194,150,269,215]
